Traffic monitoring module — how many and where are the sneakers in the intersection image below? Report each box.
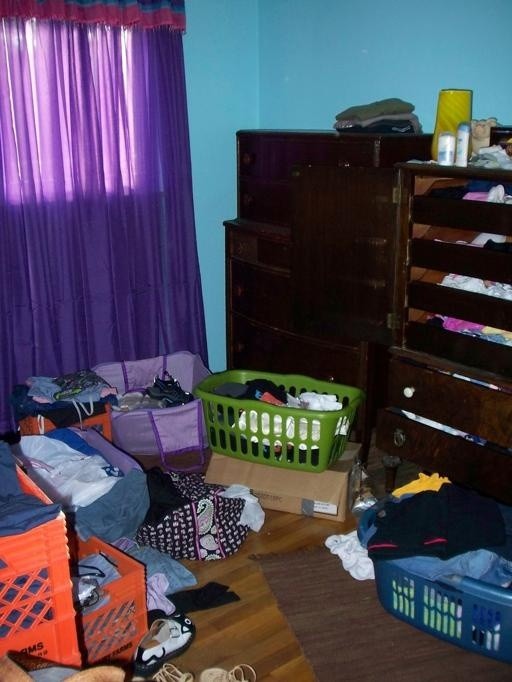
[200,663,257,682]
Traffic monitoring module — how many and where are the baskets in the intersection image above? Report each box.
[19,400,113,443]
[356,492,512,664]
[1,464,150,670]
[193,368,364,474]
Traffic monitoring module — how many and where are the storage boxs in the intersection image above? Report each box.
[204,440,363,523]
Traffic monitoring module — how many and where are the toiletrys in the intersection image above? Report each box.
[438,133,455,165]
[455,123,471,168]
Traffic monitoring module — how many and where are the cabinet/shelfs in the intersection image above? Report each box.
[376,354,512,492]
[223,220,391,447]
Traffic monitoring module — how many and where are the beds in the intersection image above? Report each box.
[381,162,512,497]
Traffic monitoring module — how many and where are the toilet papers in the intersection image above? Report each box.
[430,89,472,157]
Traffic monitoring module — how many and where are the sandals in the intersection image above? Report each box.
[132,613,195,675]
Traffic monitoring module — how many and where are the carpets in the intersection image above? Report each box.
[247,543,511,682]
